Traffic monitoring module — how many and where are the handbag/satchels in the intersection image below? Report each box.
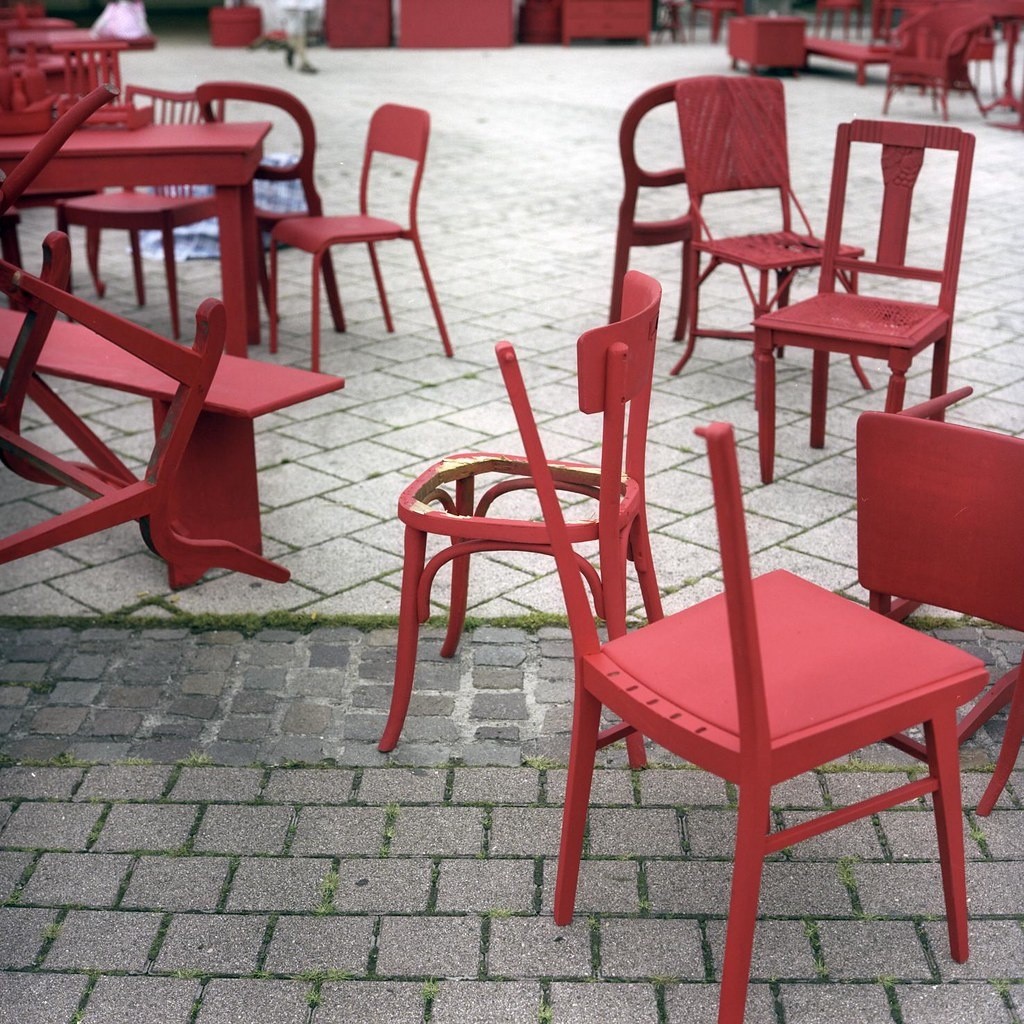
[123,154,307,262]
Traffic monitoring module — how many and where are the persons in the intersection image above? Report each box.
[656,0,685,44]
[283,0,318,73]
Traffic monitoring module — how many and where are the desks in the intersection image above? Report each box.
[0,121,274,358]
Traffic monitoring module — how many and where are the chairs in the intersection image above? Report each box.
[881,9,989,123]
[57,85,227,340]
[270,104,456,374]
[0,231,291,593]
[53,42,130,131]
[855,388,1024,759]
[378,270,664,769]
[672,79,874,394]
[748,119,974,479]
[494,340,990,1024]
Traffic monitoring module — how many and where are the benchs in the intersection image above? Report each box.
[0,307,346,557]
[806,36,893,85]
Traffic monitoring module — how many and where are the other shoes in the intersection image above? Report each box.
[300,62,317,73]
[284,44,293,66]
